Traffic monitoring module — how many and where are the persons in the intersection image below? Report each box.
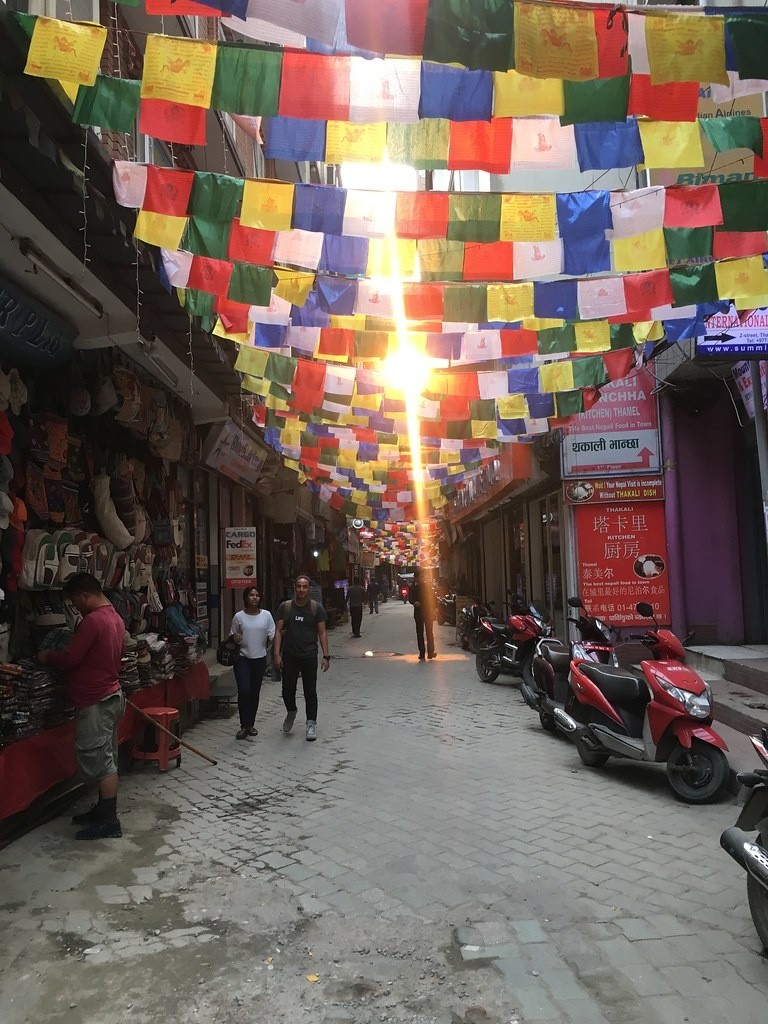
[274,574,331,741]
[344,576,366,637]
[380,577,390,602]
[366,576,381,614]
[37,573,127,842]
[408,569,438,659]
[224,584,276,740]
[398,578,410,589]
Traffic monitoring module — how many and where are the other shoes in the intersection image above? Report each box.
[376,612,378,614]
[370,611,373,614]
[418,655,425,659]
[428,653,437,659]
[352,632,360,637]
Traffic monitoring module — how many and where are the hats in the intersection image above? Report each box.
[413,571,424,577]
[0,368,29,531]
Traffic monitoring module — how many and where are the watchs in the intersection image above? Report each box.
[323,655,330,661]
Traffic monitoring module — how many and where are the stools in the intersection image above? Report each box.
[133,706,182,771]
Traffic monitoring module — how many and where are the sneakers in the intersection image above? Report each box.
[236,727,258,739]
[283,708,297,732]
[71,803,100,824]
[76,818,123,839]
[306,720,317,740]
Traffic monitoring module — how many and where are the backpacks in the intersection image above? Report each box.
[23,361,183,637]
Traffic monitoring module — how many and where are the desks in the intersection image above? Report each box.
[0,659,211,847]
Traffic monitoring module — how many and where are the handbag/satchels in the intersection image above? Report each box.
[216,634,241,666]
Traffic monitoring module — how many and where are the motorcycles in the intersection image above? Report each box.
[435,589,464,626]
[455,588,564,690]
[553,600,731,803]
[720,725,768,948]
[518,595,623,736]
[400,586,409,604]
